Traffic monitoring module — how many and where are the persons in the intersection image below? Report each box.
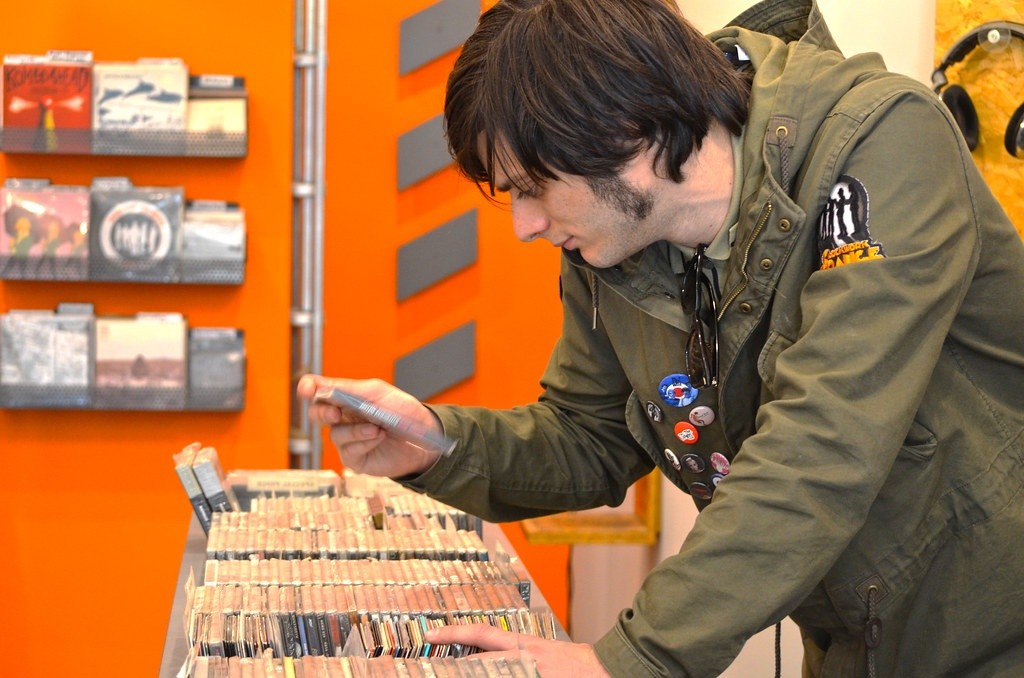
[295,0,1024,678]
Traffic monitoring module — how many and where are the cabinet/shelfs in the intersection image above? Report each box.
[0,125,248,410]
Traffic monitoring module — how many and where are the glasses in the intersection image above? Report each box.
[681,244,722,388]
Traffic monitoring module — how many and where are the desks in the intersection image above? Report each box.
[161,509,574,678]
